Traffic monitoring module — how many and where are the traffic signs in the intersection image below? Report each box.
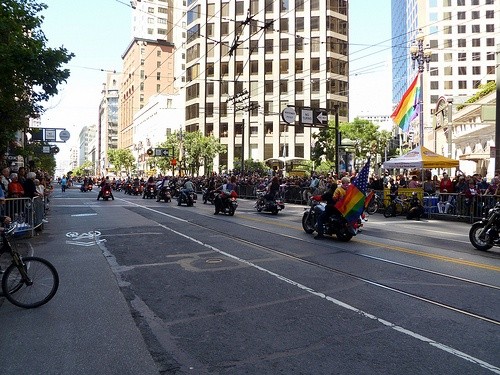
[315,110,328,125]
[162,148,169,156]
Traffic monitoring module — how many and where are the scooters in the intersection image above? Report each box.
[57,179,101,192]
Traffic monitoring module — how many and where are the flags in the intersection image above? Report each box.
[391,76,419,134]
[335,156,370,226]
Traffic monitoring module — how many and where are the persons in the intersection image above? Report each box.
[0,165,54,237]
[80,177,93,192]
[97,176,114,201]
[58,175,73,192]
[112,169,500,240]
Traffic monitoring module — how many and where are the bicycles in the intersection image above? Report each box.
[366,192,424,220]
[0,220,59,309]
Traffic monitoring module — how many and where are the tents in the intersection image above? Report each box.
[384,146,459,189]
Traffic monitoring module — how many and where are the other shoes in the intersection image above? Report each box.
[314,234,324,240]
[42,218,48,223]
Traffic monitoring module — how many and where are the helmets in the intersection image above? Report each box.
[411,192,417,196]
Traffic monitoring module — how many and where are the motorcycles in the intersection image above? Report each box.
[141,184,198,207]
[200,185,218,204]
[110,181,145,195]
[97,185,111,201]
[210,190,239,216]
[302,198,365,242]
[469,201,500,251]
[256,191,285,214]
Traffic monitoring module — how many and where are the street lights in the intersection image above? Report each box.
[409,28,432,147]
